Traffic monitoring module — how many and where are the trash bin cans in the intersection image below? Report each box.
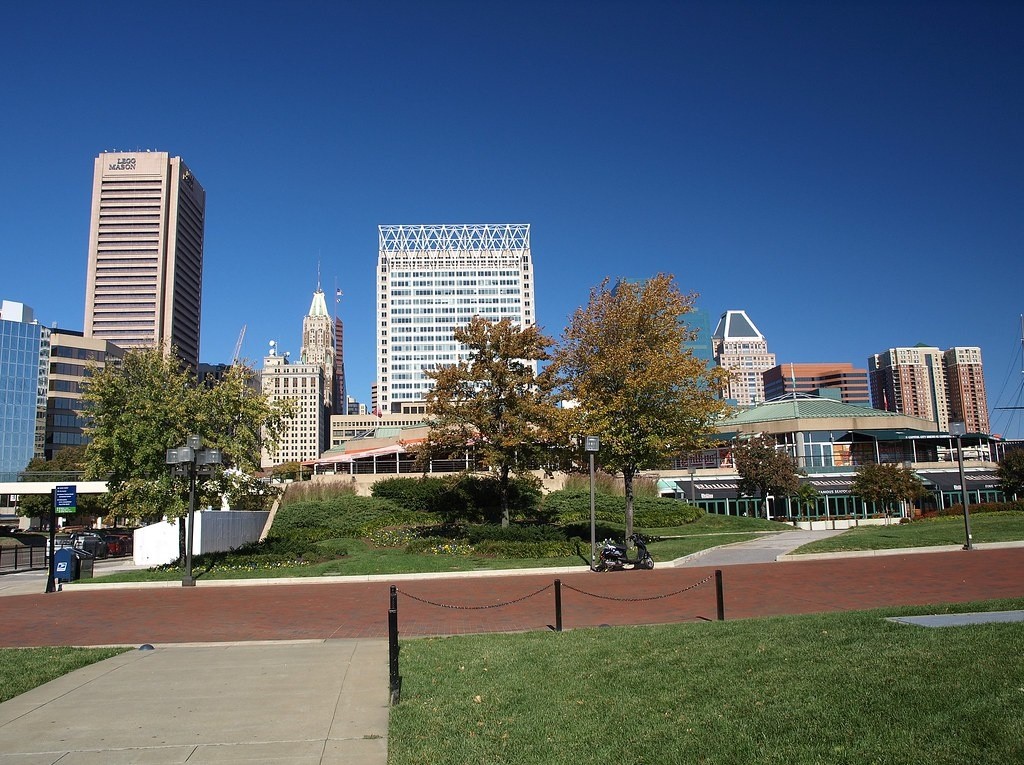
[54,548,94,582]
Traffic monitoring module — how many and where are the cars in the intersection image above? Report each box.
[46,533,133,560]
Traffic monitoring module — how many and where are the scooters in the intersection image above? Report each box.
[595,533,654,573]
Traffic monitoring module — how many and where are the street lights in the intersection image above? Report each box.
[585,436,599,571]
[166,435,221,586]
[903,460,914,517]
[948,419,972,550]
[688,466,696,507]
